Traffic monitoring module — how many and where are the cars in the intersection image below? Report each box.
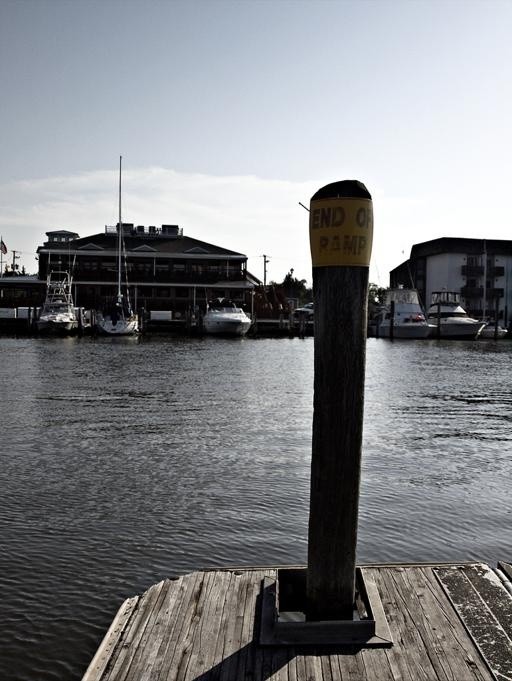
[293,302,314,319]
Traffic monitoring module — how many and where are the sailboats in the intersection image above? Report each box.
[99,156,139,335]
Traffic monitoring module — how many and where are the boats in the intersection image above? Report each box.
[203,298,250,336]
[37,271,78,332]
[425,291,480,336]
[480,325,507,339]
[372,288,437,338]
